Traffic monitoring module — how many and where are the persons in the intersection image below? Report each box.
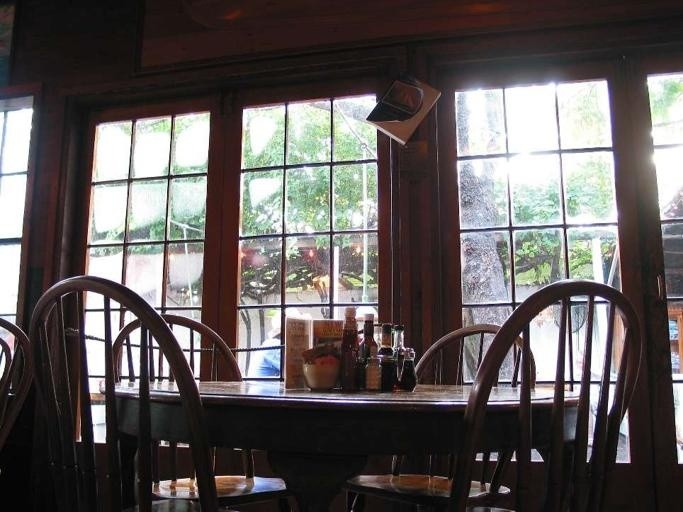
[355,306,379,337]
[527,306,580,383]
[247,306,311,378]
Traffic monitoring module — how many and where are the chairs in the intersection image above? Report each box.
[35,274,221,511]
[345,323,537,510]
[448,280,643,511]
[1,316,33,444]
[110,312,289,509]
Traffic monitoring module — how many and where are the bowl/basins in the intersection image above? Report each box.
[301,362,339,390]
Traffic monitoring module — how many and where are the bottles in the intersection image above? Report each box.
[378,322,393,367]
[357,312,377,390]
[396,347,417,392]
[339,307,360,393]
[392,323,405,386]
[365,357,382,392]
[380,357,396,393]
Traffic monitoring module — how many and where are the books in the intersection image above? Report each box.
[312,318,344,346]
[285,316,310,389]
